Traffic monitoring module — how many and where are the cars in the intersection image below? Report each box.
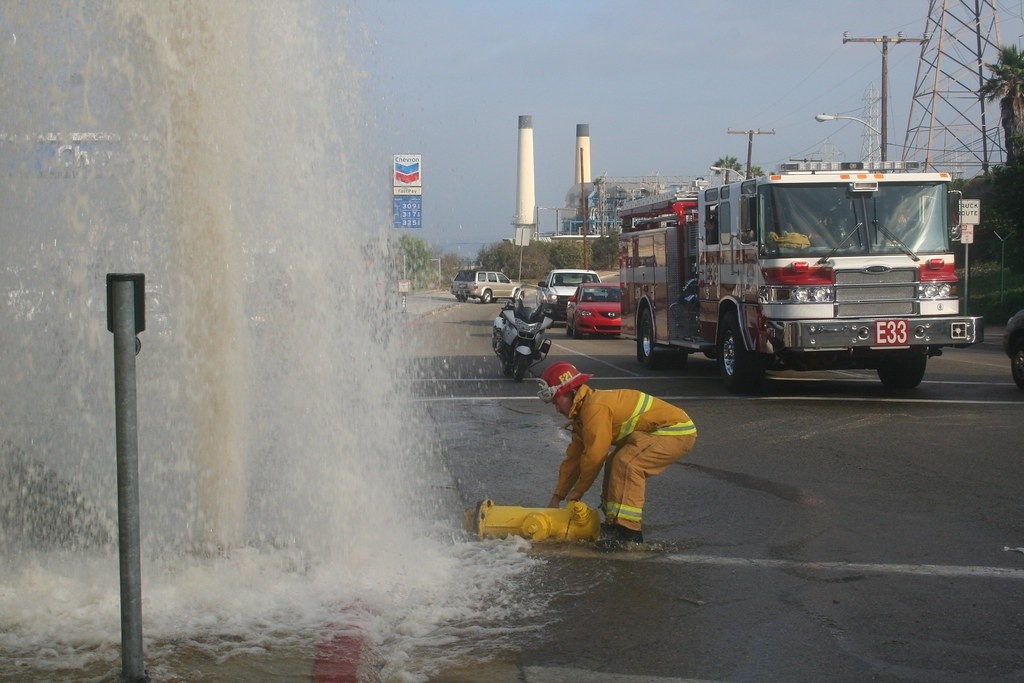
[1001,309,1024,388]
[565,283,623,340]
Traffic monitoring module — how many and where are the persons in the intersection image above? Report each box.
[538,362,697,551]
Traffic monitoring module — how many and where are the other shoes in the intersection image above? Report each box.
[595,523,644,552]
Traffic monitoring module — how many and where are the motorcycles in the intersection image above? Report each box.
[494,282,558,382]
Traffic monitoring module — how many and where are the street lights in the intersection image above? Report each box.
[816,113,887,162]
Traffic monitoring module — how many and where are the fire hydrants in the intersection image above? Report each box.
[476,495,602,543]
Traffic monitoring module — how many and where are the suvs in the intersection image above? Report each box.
[449,268,524,305]
[537,269,603,329]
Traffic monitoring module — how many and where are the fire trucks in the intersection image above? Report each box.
[617,158,985,390]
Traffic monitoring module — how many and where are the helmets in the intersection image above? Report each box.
[536,362,594,404]
